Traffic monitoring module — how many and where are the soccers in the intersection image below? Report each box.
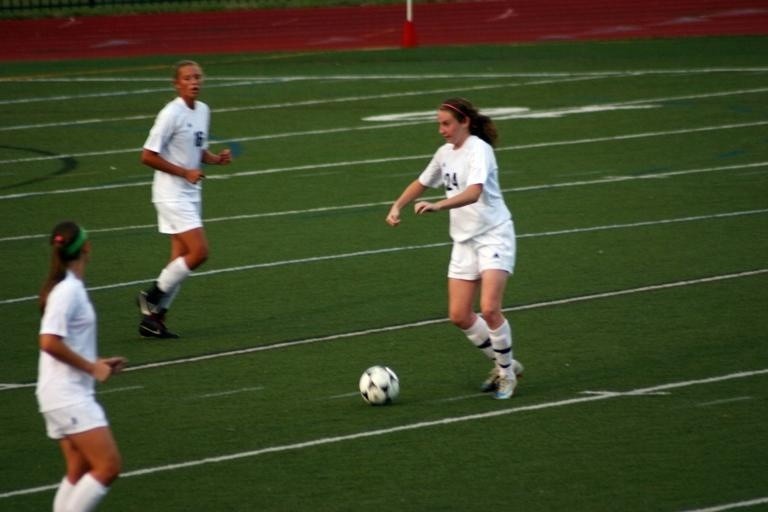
[359,364,400,405]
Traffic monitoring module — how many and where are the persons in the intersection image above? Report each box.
[33,219,130,512]
[383,95,524,401]
[135,60,232,340]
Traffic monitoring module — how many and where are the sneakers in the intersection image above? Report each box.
[138,319,180,339]
[134,287,167,322]
[492,375,518,400]
[479,358,525,393]
